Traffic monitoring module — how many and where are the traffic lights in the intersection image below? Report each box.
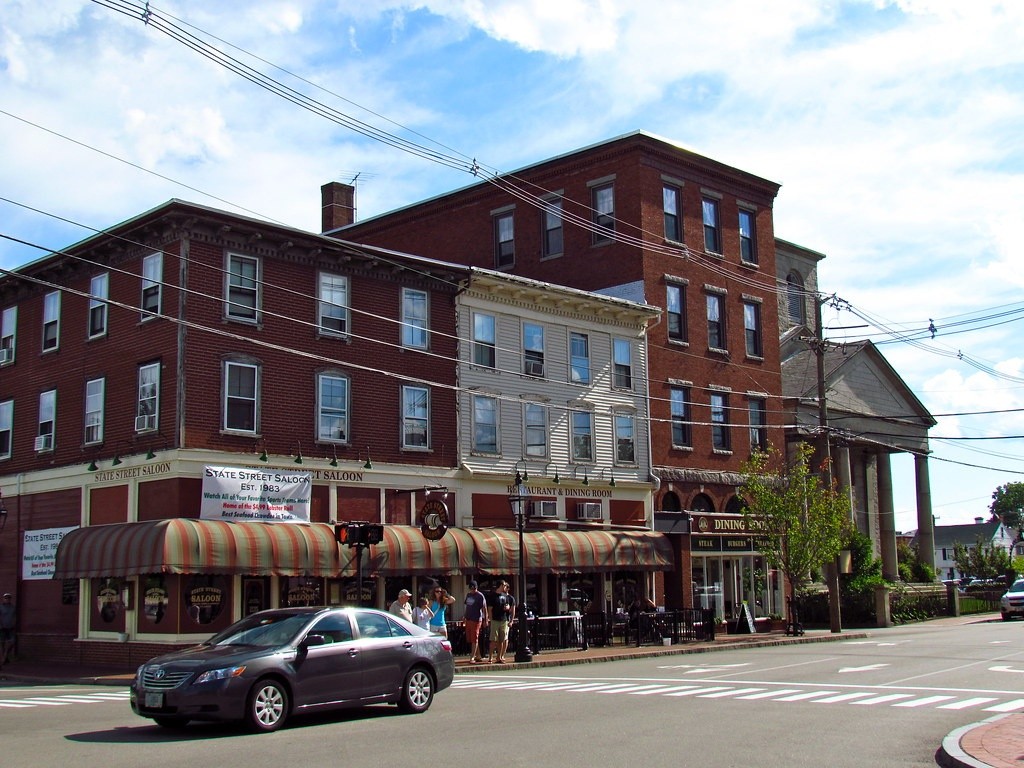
[360,524,384,543]
[334,523,359,545]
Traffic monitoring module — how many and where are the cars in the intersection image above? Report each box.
[955,573,1024,592]
[567,588,590,611]
[128,604,457,734]
[999,578,1024,622]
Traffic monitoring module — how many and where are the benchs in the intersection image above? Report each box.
[359,623,397,638]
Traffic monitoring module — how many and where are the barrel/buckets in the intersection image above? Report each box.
[663,638,671,646]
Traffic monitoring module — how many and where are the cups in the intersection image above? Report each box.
[617,608,623,613]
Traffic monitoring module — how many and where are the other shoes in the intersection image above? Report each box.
[476,658,482,662]
[470,660,475,664]
[487,659,492,664]
[501,658,505,661]
[495,661,505,664]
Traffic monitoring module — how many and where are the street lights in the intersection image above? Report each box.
[506,469,531,662]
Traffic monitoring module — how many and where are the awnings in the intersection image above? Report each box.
[53,516,678,581]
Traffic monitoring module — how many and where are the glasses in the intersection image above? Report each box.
[434,591,443,593]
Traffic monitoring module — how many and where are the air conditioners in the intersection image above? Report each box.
[34,436,51,451]
[134,415,155,431]
[531,501,558,517]
[527,361,544,376]
[0,348,13,363]
[577,503,601,519]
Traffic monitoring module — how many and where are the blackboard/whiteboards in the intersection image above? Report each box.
[735,603,757,634]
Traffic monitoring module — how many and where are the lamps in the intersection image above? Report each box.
[252,438,373,470]
[86,433,167,473]
[515,459,616,487]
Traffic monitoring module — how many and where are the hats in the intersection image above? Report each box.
[469,581,478,590]
[399,589,412,596]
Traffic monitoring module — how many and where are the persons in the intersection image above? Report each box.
[0,593,17,666]
[389,587,456,639]
[628,595,663,644]
[462,579,516,665]
